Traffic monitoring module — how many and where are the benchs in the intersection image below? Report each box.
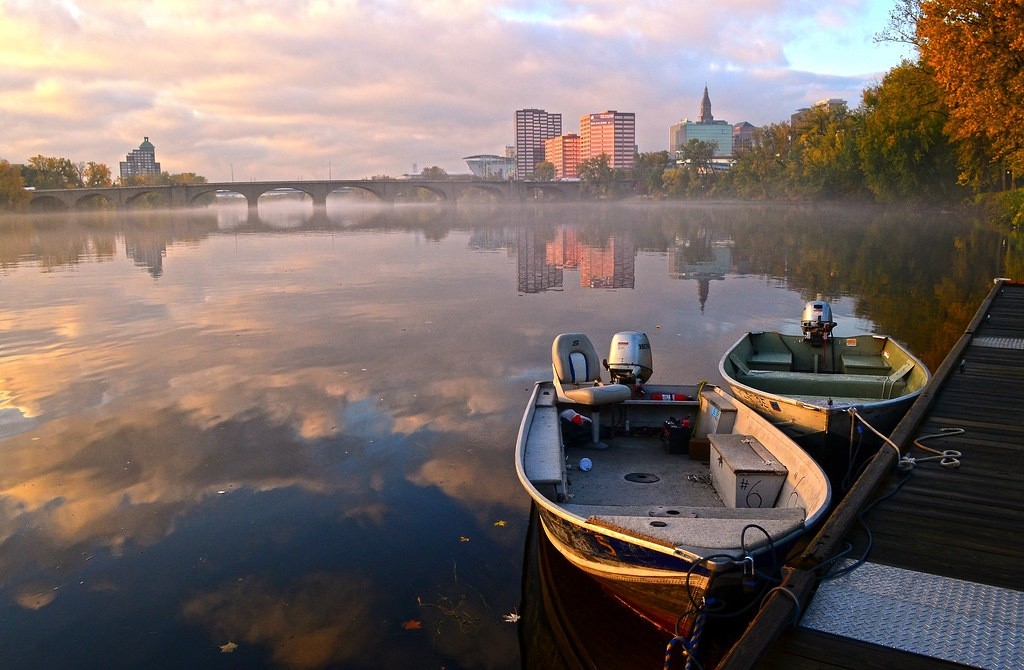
[735,369,905,399]
[524,387,788,509]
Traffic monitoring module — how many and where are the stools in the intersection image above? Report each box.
[747,351,792,372]
[840,354,891,376]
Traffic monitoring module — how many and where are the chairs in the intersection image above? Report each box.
[551,334,631,450]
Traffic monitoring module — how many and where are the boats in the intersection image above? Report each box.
[720,298,933,445]
[514,328,834,652]
